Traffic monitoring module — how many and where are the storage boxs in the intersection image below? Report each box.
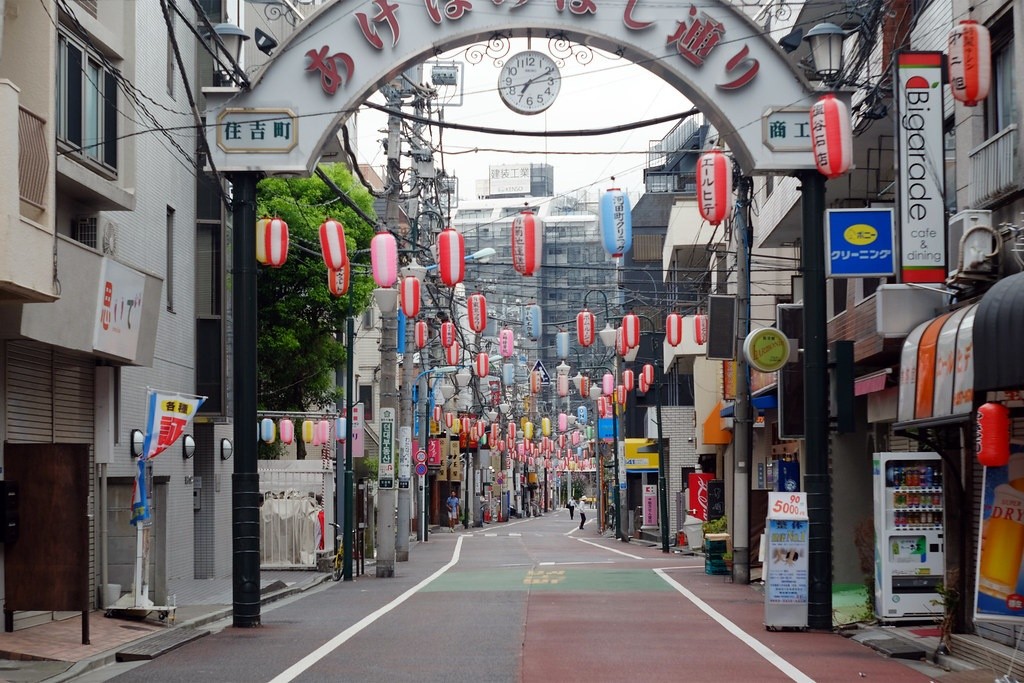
[704,533,733,575]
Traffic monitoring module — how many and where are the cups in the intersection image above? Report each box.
[979,492,1024,599]
[1009,453,1024,492]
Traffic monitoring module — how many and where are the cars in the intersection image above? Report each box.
[510,505,517,516]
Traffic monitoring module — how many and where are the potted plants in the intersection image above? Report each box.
[701,514,728,572]
[930,583,960,648]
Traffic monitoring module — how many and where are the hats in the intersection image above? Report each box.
[581,496,587,501]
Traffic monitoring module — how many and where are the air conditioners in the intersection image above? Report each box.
[78,215,118,256]
[948,209,992,279]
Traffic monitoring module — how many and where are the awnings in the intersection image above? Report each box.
[972,271,1024,391]
[892,301,980,431]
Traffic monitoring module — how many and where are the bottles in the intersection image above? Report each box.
[893,466,941,531]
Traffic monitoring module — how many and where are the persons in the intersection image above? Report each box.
[579,495,587,530]
[446,489,460,532]
[569,496,578,520]
[609,502,616,530]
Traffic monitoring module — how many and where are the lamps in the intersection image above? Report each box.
[203,22,250,86]
[183,434,195,460]
[221,438,232,460]
[131,429,144,457]
[802,23,846,81]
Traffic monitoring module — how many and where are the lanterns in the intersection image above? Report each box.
[328,257,350,296]
[599,187,632,257]
[512,211,543,276]
[320,218,347,272]
[371,231,398,288]
[265,217,289,269]
[257,277,707,474]
[810,93,852,178]
[948,19,992,106]
[437,227,464,286]
[971,401,1011,466]
[256,217,271,265]
[696,150,732,225]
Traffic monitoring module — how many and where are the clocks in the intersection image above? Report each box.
[498,50,562,115]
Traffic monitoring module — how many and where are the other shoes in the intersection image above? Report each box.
[449,528,455,533]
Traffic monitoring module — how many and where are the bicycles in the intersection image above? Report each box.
[328,522,357,581]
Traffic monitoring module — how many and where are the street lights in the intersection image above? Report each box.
[599,315,669,553]
[395,366,458,562]
[374,246,497,572]
[464,404,510,526]
[574,366,621,539]
